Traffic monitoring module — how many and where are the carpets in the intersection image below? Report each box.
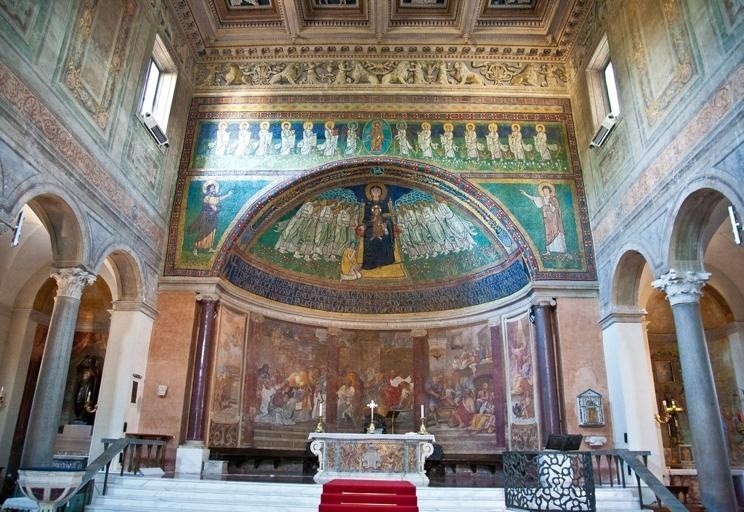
[317,479,419,511]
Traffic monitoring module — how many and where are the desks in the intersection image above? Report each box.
[123,432,175,471]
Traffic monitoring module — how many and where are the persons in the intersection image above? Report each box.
[255,363,330,423]
[335,368,415,424]
[720,404,744,461]
[273,181,477,282]
[519,180,568,257]
[187,180,233,256]
[423,342,497,434]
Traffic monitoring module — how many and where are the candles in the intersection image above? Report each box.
[420,404,425,418]
[371,404,374,422]
[319,402,323,416]
